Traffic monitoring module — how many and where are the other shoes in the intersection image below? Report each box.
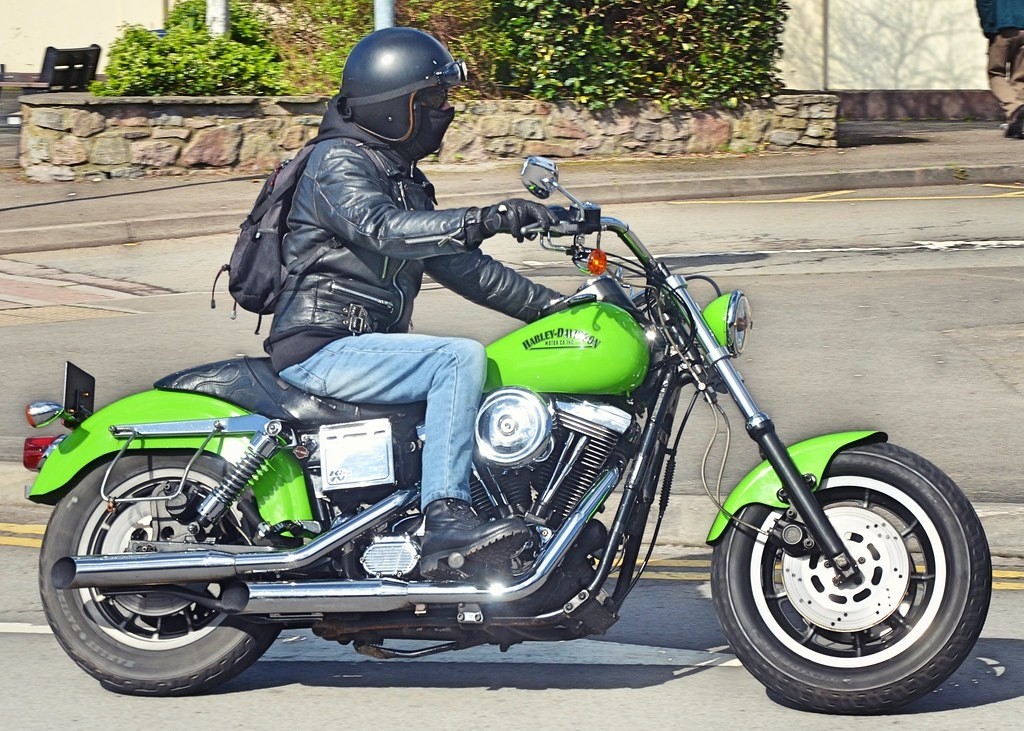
[1005,105,1024,137]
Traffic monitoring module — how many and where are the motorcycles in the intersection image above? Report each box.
[14,160,994,712]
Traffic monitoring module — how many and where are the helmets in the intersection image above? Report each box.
[341,27,459,143]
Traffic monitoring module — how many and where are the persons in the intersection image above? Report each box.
[976,0,1024,140]
[261,25,570,582]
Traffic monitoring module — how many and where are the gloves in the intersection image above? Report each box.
[481,197,560,243]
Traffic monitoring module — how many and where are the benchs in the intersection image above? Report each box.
[21,44,101,95]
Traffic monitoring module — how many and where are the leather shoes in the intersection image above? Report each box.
[420,499,527,578]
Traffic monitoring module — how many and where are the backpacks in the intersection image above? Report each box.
[227,135,384,315]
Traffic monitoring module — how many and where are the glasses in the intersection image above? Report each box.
[347,59,469,107]
